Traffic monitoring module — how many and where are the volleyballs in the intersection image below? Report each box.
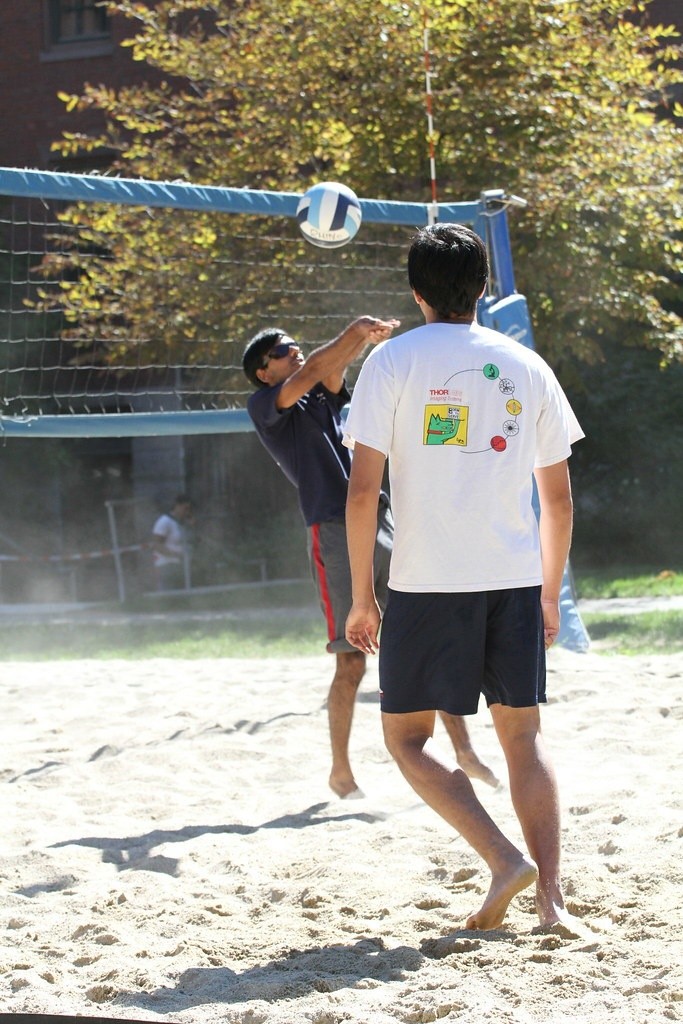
[296,181,362,249]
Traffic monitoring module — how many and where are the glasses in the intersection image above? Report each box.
[263,341,300,368]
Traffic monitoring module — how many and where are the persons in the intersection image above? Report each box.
[236,315,503,804]
[340,224,586,927]
[150,495,215,591]
